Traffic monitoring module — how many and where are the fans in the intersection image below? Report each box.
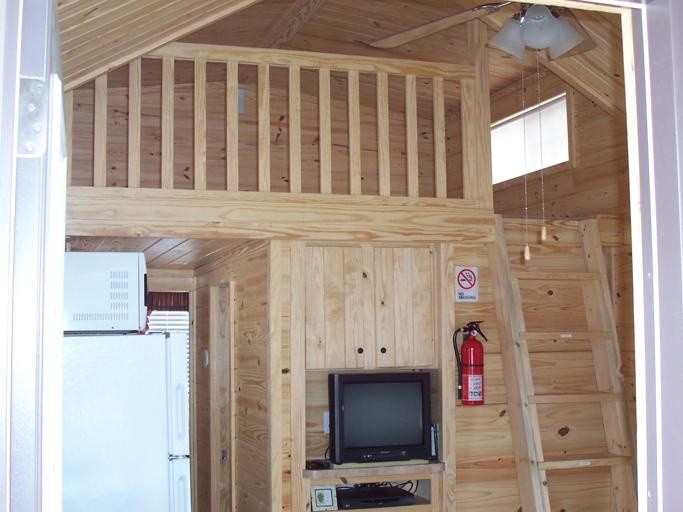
[367,4,512,56]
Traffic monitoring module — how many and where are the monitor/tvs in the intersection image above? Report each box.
[328,372,432,465]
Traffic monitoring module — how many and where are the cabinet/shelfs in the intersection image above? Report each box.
[287,243,455,511]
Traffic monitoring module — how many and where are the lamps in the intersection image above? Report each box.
[484,1,585,63]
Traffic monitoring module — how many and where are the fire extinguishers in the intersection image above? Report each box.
[453,320,488,406]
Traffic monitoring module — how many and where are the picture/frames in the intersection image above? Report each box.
[310,484,337,511]
